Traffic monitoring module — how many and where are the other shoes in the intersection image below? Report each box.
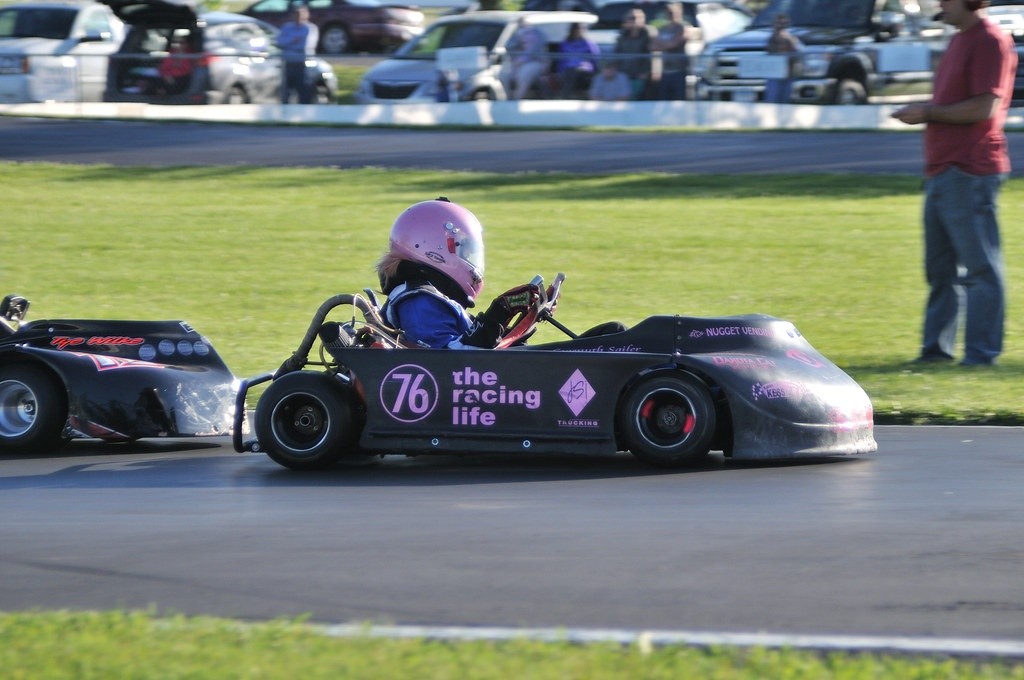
[957,358,991,366]
[904,355,945,367]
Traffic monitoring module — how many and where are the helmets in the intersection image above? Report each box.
[389,196,485,308]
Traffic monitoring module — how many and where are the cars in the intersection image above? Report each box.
[980,7,1024,97]
[348,2,754,104]
[1,0,338,107]
[240,0,424,56]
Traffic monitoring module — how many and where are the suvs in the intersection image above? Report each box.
[686,1,923,105]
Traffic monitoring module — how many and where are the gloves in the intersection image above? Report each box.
[537,285,562,321]
[486,283,541,328]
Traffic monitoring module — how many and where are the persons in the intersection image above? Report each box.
[379,197,559,349]
[889,0,1018,364]
[160,37,193,94]
[273,7,319,104]
[432,0,805,102]
